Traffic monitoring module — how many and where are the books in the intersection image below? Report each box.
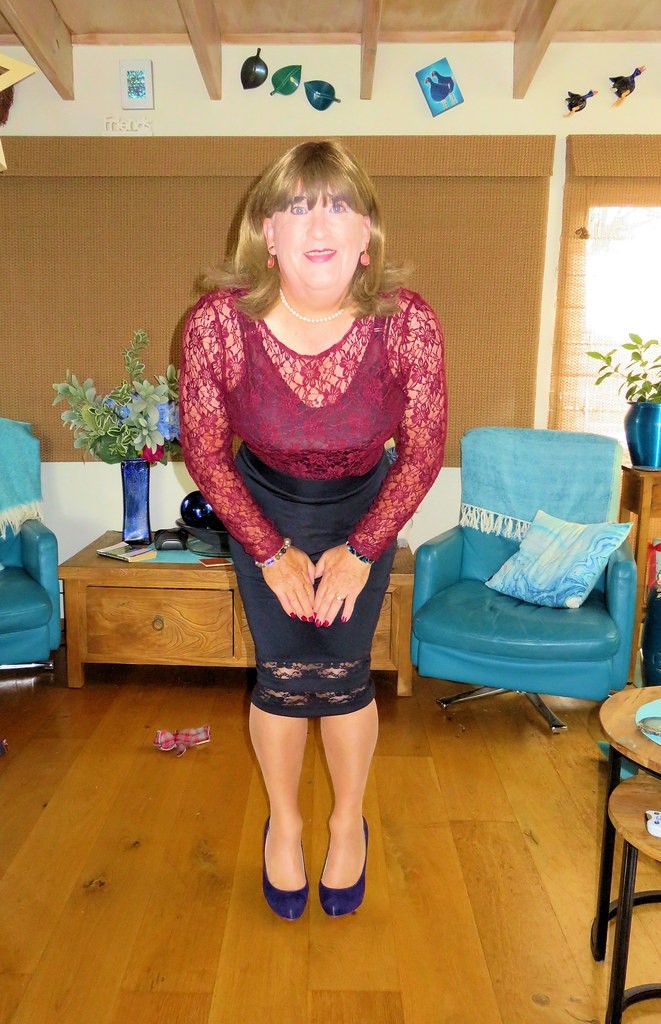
[198,557,233,568]
[96,541,156,563]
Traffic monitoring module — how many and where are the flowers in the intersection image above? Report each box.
[53,329,184,466]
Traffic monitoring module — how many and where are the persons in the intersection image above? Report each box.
[168,139,451,922]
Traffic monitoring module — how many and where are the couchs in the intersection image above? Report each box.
[0,418,62,672]
[407,426,640,734]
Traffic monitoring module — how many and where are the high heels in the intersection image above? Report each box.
[319,815,368,916]
[263,814,309,921]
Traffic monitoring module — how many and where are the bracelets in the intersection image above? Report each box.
[254,537,291,568]
[344,539,375,565]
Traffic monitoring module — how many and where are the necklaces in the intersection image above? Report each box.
[278,287,349,324]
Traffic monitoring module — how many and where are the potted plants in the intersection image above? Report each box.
[586,333,661,471]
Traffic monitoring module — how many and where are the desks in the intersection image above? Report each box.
[57,529,415,697]
[589,685,661,964]
[606,774,661,1024]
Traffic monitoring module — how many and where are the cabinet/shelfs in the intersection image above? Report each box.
[243,586,391,663]
[617,464,661,680]
[85,583,235,660]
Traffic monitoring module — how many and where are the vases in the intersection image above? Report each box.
[119,458,152,546]
